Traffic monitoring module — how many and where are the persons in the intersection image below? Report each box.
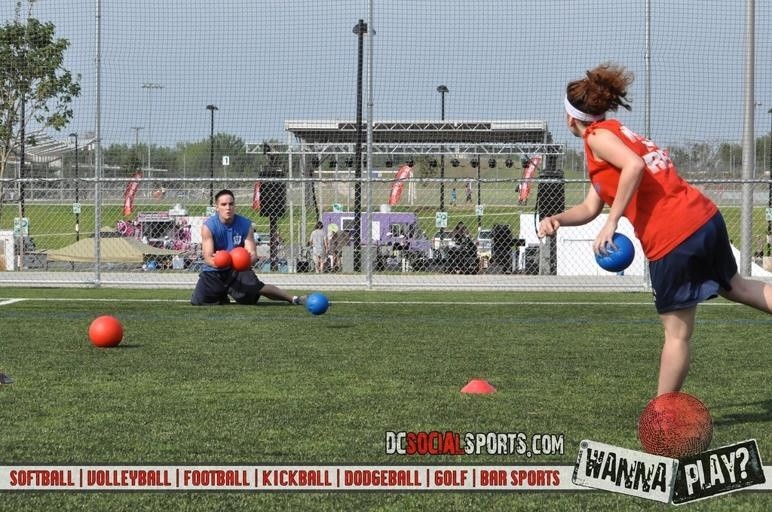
[451,188,457,206]
[309,221,328,273]
[401,237,409,272]
[161,186,166,198]
[191,189,308,306]
[328,230,337,271]
[452,221,465,244]
[538,60,772,396]
[464,184,472,201]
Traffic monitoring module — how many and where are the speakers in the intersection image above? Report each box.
[260,167,286,217]
[539,172,565,221]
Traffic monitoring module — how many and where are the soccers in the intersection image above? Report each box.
[229,247,251,271]
[214,250,230,269]
[639,392,713,459]
[595,233,634,272]
[306,293,329,315]
[88,315,122,347]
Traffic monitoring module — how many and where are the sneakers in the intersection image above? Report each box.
[294,296,307,305]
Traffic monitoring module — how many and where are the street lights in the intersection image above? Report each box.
[68,132,80,241]
[131,126,145,166]
[766,106,772,258]
[351,18,377,273]
[205,103,219,205]
[140,83,164,197]
[436,85,449,246]
[16,79,30,219]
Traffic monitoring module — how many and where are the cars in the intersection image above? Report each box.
[254,232,286,262]
[432,229,461,252]
[476,229,494,252]
